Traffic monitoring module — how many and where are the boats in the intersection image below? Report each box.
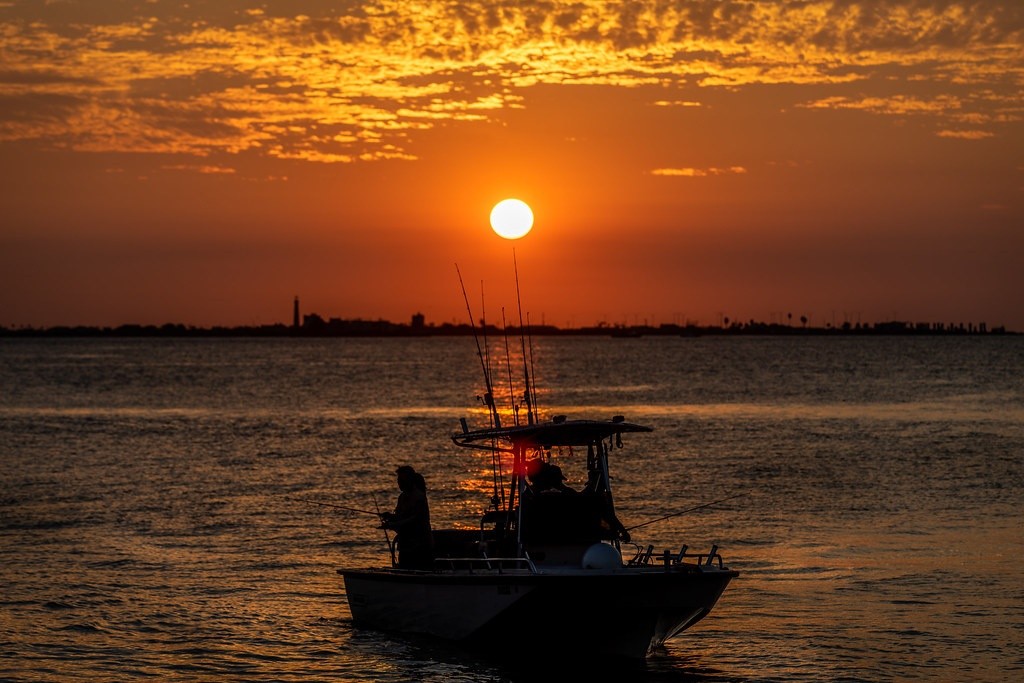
[337,247,739,658]
